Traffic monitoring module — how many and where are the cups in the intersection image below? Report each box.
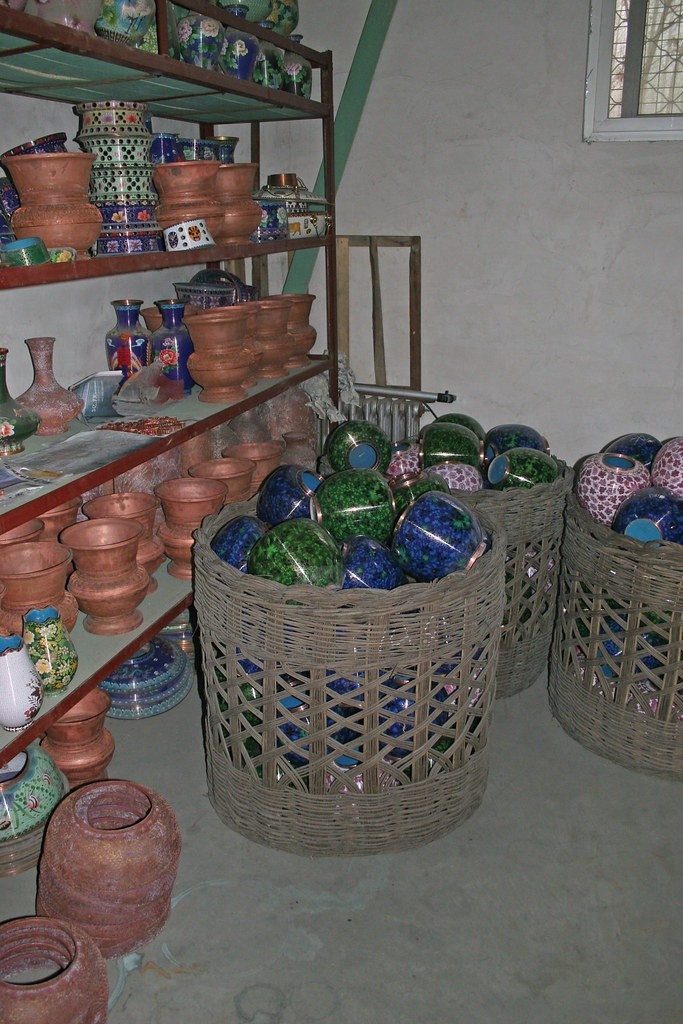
[22,606,79,695]
[0,635,45,732]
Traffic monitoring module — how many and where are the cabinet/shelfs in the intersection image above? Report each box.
[0,0,342,1024]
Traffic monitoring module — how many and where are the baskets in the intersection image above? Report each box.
[192,456,682,858]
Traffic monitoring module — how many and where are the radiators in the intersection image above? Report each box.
[314,391,430,458]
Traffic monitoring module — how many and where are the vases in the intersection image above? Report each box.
[0,336,85,457]
[0,603,79,732]
[105,298,199,396]
[175,0,313,100]
